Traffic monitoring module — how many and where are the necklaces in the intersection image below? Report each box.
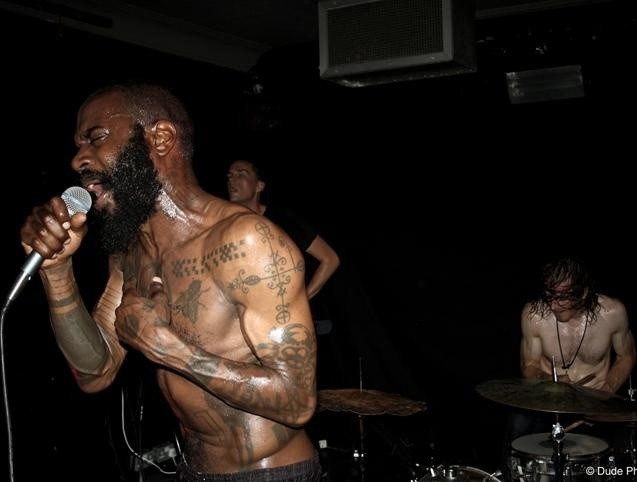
[556,306,589,369]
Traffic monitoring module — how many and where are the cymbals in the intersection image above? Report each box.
[316,390,426,416]
[476,379,636,416]
[512,432,608,456]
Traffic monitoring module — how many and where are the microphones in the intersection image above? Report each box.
[6,186,92,303]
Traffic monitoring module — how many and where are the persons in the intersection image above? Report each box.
[498,254,636,464]
[19,79,329,482]
[226,150,341,302]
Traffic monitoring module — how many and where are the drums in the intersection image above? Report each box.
[407,464,500,482]
[509,455,600,482]
